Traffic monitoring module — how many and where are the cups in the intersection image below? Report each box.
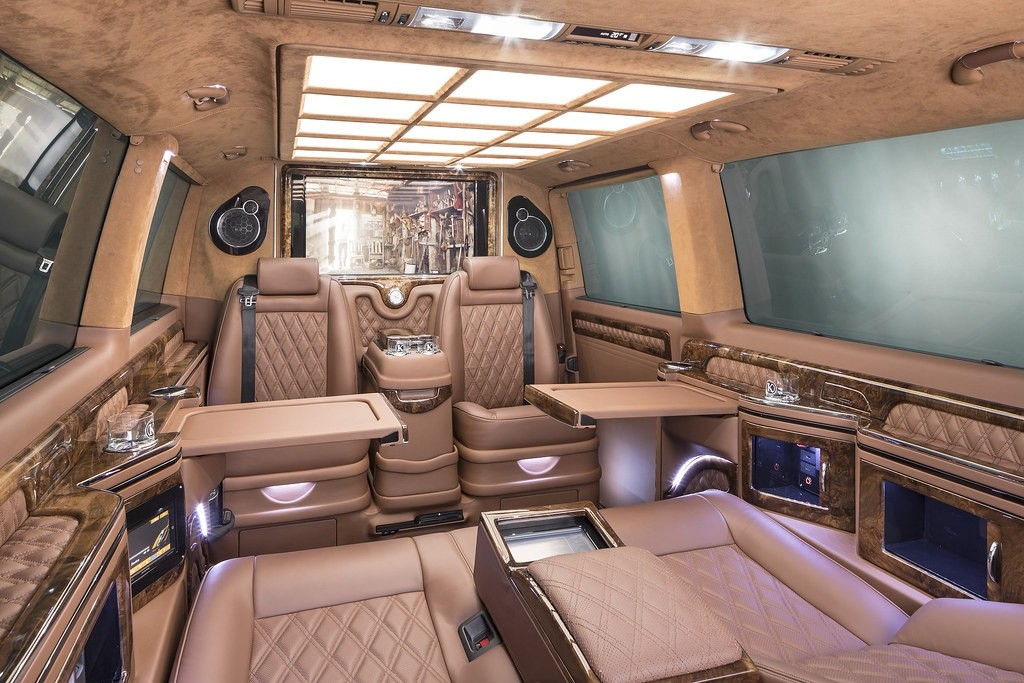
[386,335,440,355]
[765,373,800,402]
[106,411,155,451]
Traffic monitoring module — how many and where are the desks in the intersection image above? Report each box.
[524,380,741,429]
[160,390,411,459]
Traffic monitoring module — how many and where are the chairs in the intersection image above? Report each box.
[169,253,1024,682]
